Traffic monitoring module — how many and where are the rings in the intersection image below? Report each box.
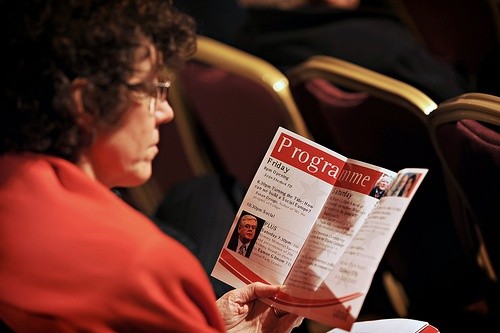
[274,307,281,317]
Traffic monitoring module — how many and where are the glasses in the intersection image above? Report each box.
[122,80,170,102]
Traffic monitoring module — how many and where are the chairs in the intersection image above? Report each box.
[119,33,500,333]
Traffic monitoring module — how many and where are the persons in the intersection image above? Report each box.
[391,175,408,196]
[167,0,500,132]
[369,176,390,199]
[402,174,416,197]
[0,0,304,333]
[231,215,257,258]
[157,176,236,300]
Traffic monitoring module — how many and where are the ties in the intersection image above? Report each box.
[375,189,382,197]
[238,245,246,256]
